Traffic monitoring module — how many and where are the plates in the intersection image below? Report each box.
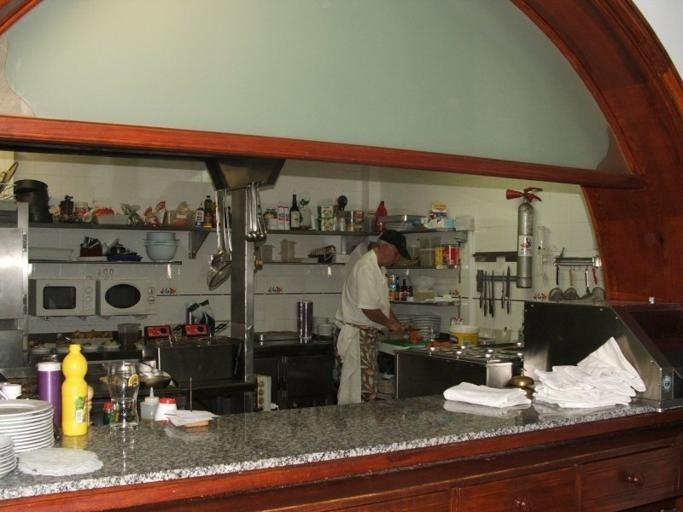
[0,399,55,479]
[312,315,337,340]
[30,338,142,355]
[386,222,411,231]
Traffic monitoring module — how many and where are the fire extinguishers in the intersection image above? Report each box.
[507,188,543,287]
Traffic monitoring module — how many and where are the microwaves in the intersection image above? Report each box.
[99,277,158,317]
[28,279,96,316]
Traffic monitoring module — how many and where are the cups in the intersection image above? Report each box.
[256,239,295,262]
[118,323,137,350]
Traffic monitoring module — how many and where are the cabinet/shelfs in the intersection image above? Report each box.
[15,199,476,327]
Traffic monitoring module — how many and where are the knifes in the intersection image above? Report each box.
[388,329,421,333]
[478,265,516,318]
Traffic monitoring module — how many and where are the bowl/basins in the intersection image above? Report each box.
[143,232,180,262]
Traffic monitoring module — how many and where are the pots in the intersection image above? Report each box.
[14,180,49,222]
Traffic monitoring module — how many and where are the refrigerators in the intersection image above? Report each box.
[0,202,28,371]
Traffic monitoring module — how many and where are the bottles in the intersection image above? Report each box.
[203,195,213,228]
[264,192,301,232]
[396,275,409,304]
[37,344,90,436]
[393,310,441,339]
[375,201,387,231]
[107,359,179,430]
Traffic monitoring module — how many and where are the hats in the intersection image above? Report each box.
[379,229,412,260]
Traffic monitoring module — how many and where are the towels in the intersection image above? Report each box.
[442,335,646,409]
[443,401,532,419]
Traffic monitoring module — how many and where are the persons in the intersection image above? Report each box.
[328,229,412,405]
